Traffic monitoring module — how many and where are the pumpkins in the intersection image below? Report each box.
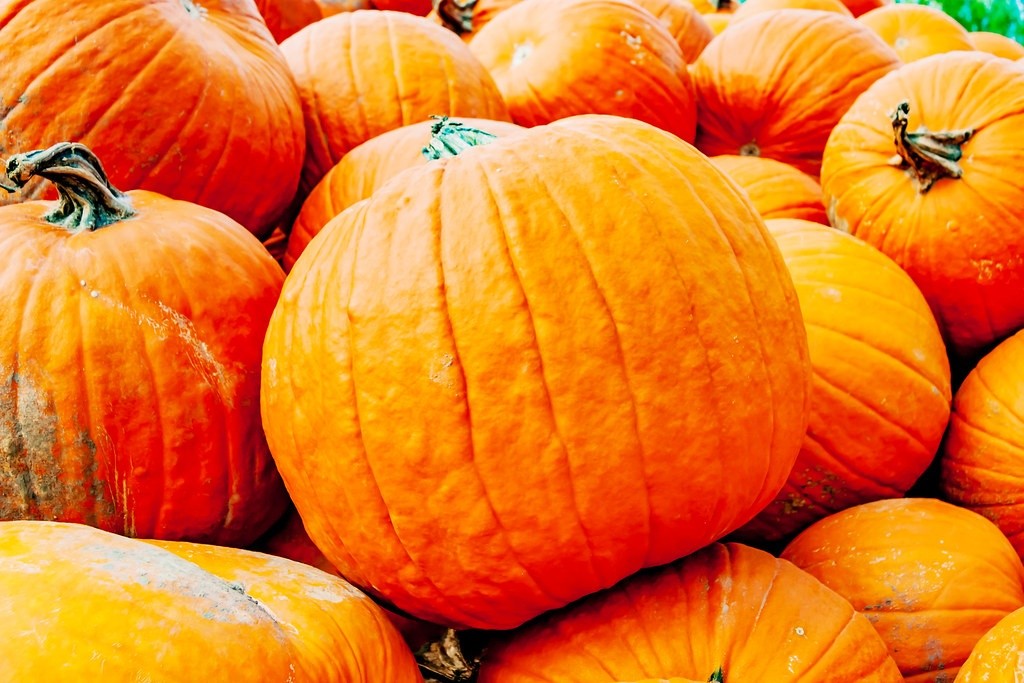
[1,2,1024,682]
